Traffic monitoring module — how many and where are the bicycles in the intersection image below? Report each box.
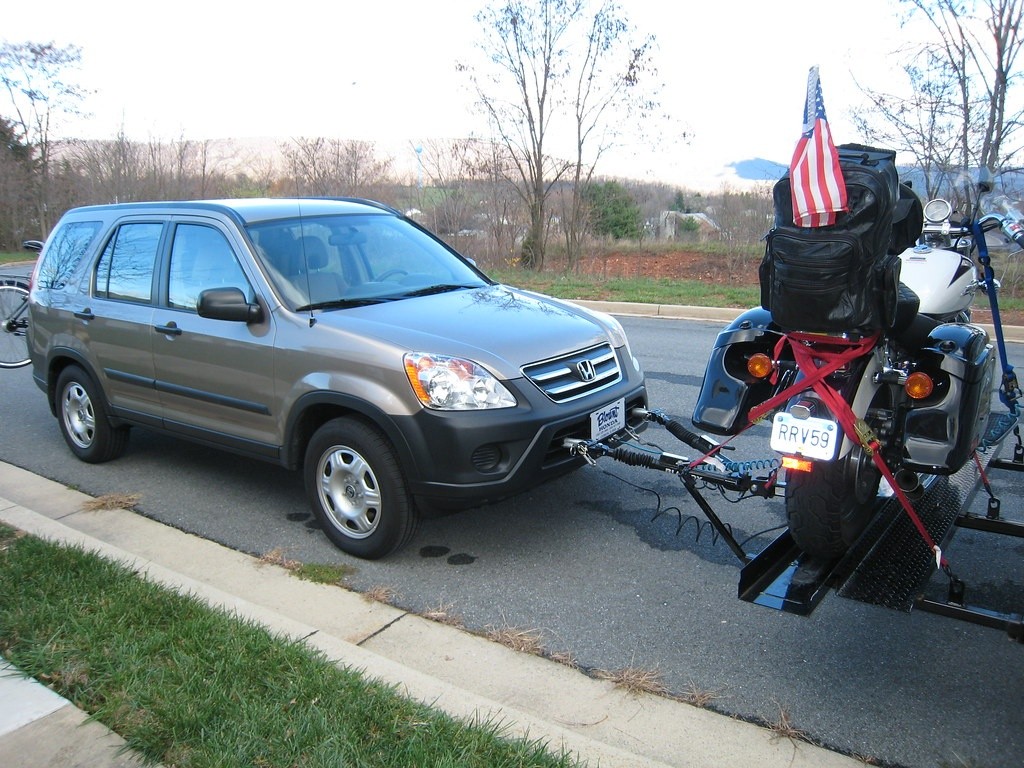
[1,239,45,369]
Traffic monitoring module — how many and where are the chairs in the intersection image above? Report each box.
[291,236,348,300]
[195,244,245,304]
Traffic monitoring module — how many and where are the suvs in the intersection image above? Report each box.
[25,198,650,562]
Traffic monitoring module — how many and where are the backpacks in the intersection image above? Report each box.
[759,163,900,334]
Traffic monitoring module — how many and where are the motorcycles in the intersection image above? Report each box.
[689,165,1024,552]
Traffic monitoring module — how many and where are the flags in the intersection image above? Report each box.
[790,67,849,228]
